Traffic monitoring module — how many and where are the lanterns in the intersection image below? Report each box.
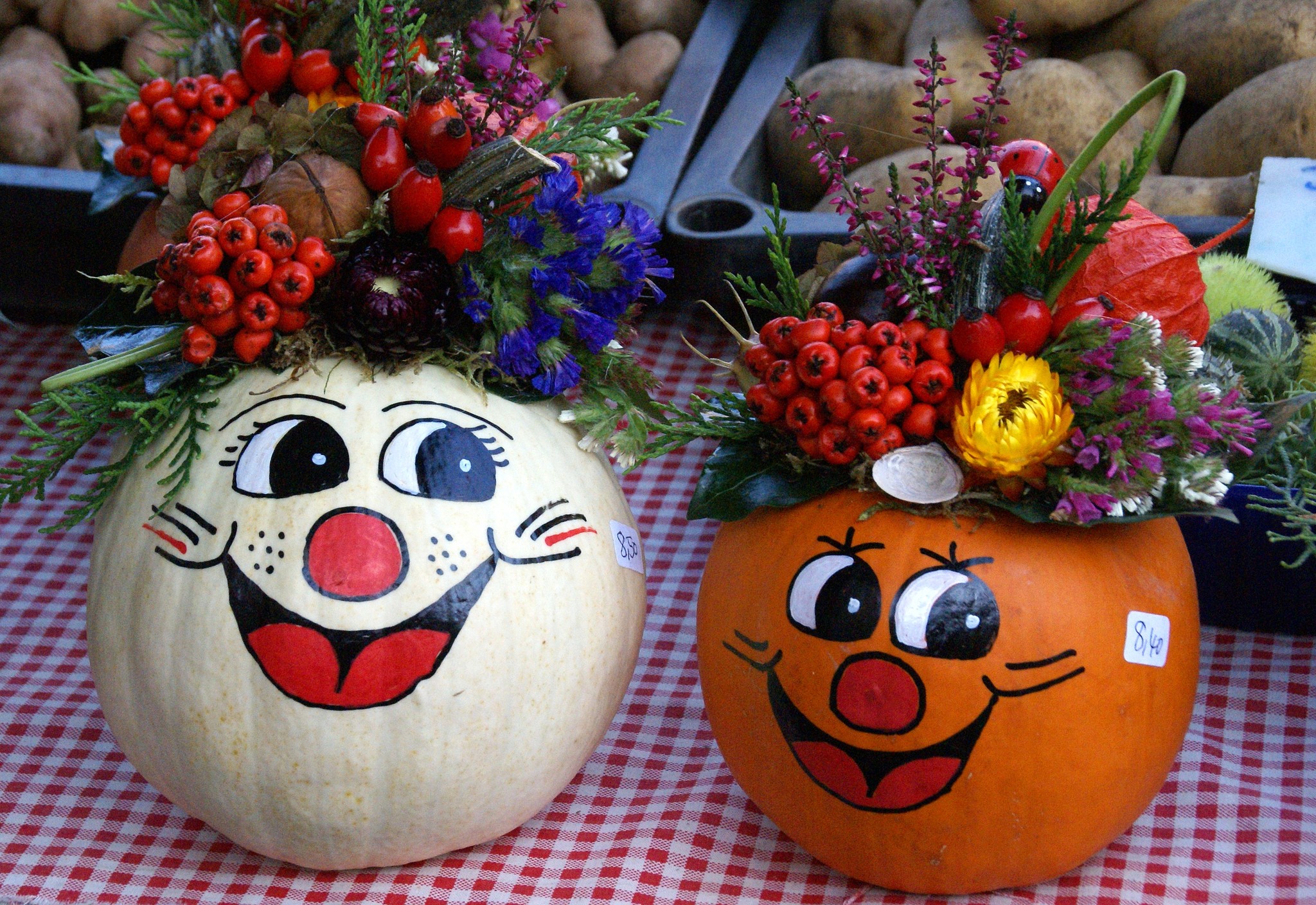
[701,478,1199,894]
[81,335,650,872]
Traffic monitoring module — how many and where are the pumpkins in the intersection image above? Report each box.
[695,490,1199,895]
[89,356,649,869]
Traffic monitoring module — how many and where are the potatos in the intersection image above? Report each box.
[0,0,1315,214]
